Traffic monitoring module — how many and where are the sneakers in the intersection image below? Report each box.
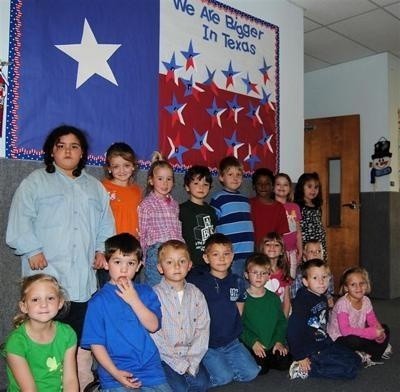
[288,361,308,380]
[355,343,392,364]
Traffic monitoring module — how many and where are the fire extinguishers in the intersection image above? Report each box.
[0,61,11,138]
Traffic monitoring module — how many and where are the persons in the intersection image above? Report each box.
[327,266,393,368]
[1,124,363,392]
